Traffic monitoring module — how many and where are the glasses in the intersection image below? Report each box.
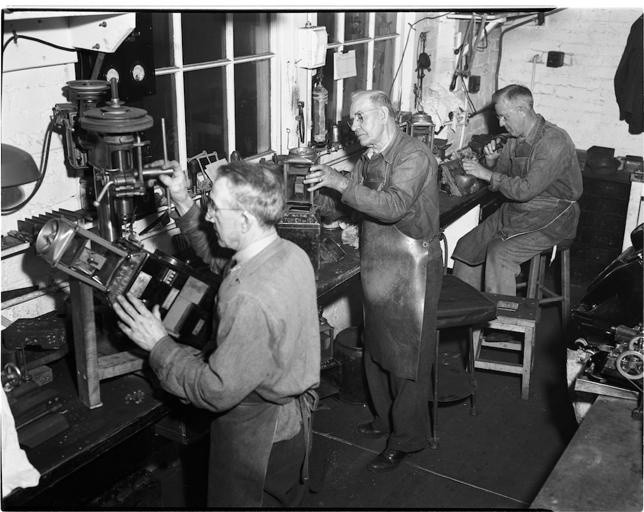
[207,202,244,217]
[346,107,380,127]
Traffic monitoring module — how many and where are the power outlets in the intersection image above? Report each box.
[528,51,542,63]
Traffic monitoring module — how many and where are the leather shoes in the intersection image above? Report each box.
[368,448,405,472]
[485,332,515,343]
[356,423,386,436]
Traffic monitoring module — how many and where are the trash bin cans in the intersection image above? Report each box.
[333,325,368,405]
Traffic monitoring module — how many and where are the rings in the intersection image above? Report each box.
[319,177,323,182]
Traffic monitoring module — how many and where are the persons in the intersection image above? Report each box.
[450,84,584,343]
[302,90,447,474]
[112,161,322,507]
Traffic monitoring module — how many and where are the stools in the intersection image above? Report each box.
[516,239,573,330]
[466,292,539,401]
[429,275,496,450]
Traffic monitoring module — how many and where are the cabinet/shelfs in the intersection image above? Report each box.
[570,170,631,286]
